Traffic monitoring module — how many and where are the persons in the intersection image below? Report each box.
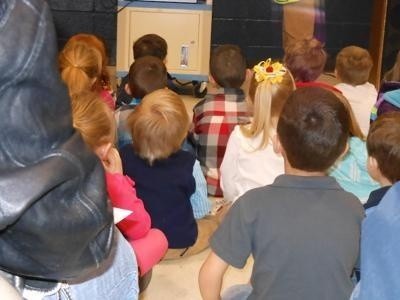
[120,87,232,260]
[0,0,139,300]
[219,61,295,223]
[115,56,194,153]
[61,33,116,108]
[327,88,400,204]
[360,109,400,300]
[193,45,254,198]
[59,43,102,96]
[334,45,378,140]
[198,85,366,300]
[117,33,184,105]
[284,36,343,95]
[69,91,169,293]
